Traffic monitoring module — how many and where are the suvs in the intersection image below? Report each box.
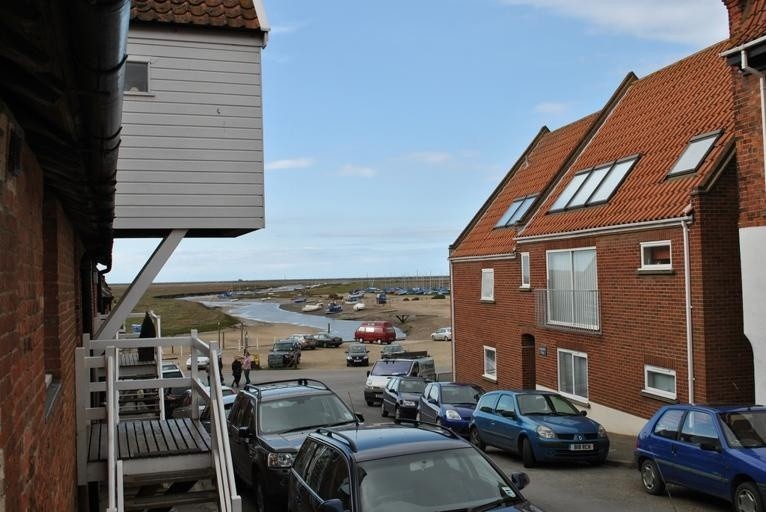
[267,341,302,369]
[219,377,367,511]
[281,413,547,511]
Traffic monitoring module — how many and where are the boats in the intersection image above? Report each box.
[216,291,235,299]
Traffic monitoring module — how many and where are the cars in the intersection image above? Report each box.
[287,283,454,368]
[161,349,241,443]
[468,389,611,468]
[633,403,766,512]
[381,373,430,429]
[412,382,491,438]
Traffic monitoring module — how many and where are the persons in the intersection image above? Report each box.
[216,355,224,386]
[231,355,243,388]
[241,351,252,384]
[205,361,211,386]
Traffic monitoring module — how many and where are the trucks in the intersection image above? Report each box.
[362,348,439,408]
